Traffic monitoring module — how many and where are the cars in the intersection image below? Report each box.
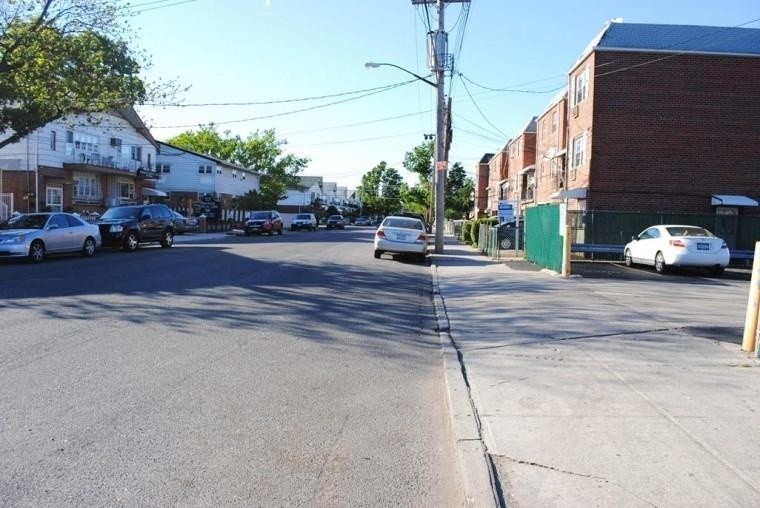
[172,211,200,235]
[374,212,428,262]
[327,214,385,230]
[624,224,730,275]
[493,220,524,249]
[0,212,102,264]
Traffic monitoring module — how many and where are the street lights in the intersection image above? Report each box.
[364,62,445,253]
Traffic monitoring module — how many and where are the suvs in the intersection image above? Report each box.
[291,213,317,232]
[94,204,177,252]
[244,210,284,236]
